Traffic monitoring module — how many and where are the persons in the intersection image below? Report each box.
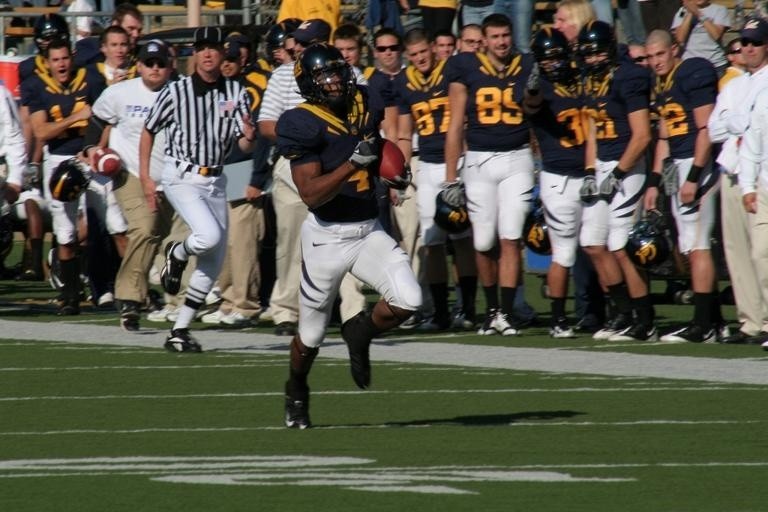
[267,19,304,64]
[257,18,373,337]
[553,1,606,333]
[455,22,485,54]
[624,37,652,66]
[194,202,231,320]
[716,38,746,95]
[147,256,197,323]
[670,1,730,79]
[418,0,460,39]
[72,26,139,304]
[709,17,768,346]
[512,268,536,321]
[0,76,25,263]
[333,23,376,80]
[138,27,256,355]
[366,0,410,39]
[72,4,143,81]
[578,20,658,344]
[17,13,71,281]
[627,41,650,68]
[225,30,271,90]
[29,40,161,316]
[444,12,540,338]
[63,0,94,56]
[645,28,733,344]
[394,28,481,334]
[528,26,618,340]
[463,0,495,26]
[88,39,187,335]
[274,43,422,429]
[373,28,431,330]
[433,32,456,61]
[199,39,267,328]
[165,43,185,81]
[737,86,768,350]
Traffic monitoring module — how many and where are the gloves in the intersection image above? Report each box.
[597,172,625,205]
[443,180,466,204]
[577,176,599,199]
[347,136,378,169]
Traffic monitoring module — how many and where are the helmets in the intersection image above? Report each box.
[626,213,680,272]
[49,157,90,203]
[293,43,358,111]
[432,190,471,235]
[33,12,70,58]
[533,28,566,69]
[266,18,304,53]
[578,20,615,71]
[522,204,554,255]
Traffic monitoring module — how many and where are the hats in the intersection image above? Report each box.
[287,18,329,43]
[136,41,169,59]
[740,18,768,39]
[192,26,222,46]
[222,37,240,60]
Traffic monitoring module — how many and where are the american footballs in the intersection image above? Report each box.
[379,138,405,182]
[94,149,123,177]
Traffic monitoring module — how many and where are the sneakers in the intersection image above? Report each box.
[167,304,192,322]
[418,315,444,332]
[120,299,143,333]
[284,381,314,432]
[275,321,299,336]
[446,313,472,331]
[19,238,44,281]
[164,327,203,354]
[662,316,718,342]
[340,312,376,389]
[56,293,81,316]
[88,292,114,309]
[577,314,604,329]
[491,309,521,335]
[475,311,494,334]
[222,309,247,325]
[594,314,632,339]
[160,240,192,294]
[48,249,79,294]
[201,310,231,324]
[400,314,427,331]
[610,319,659,342]
[547,318,574,337]
[146,303,176,322]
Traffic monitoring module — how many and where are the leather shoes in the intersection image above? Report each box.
[722,329,748,343]
[744,332,768,346]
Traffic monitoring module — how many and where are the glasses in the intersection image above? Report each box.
[728,47,741,54]
[375,44,400,53]
[141,59,170,72]
[741,38,765,47]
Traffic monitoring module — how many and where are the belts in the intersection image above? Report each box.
[175,161,223,178]
